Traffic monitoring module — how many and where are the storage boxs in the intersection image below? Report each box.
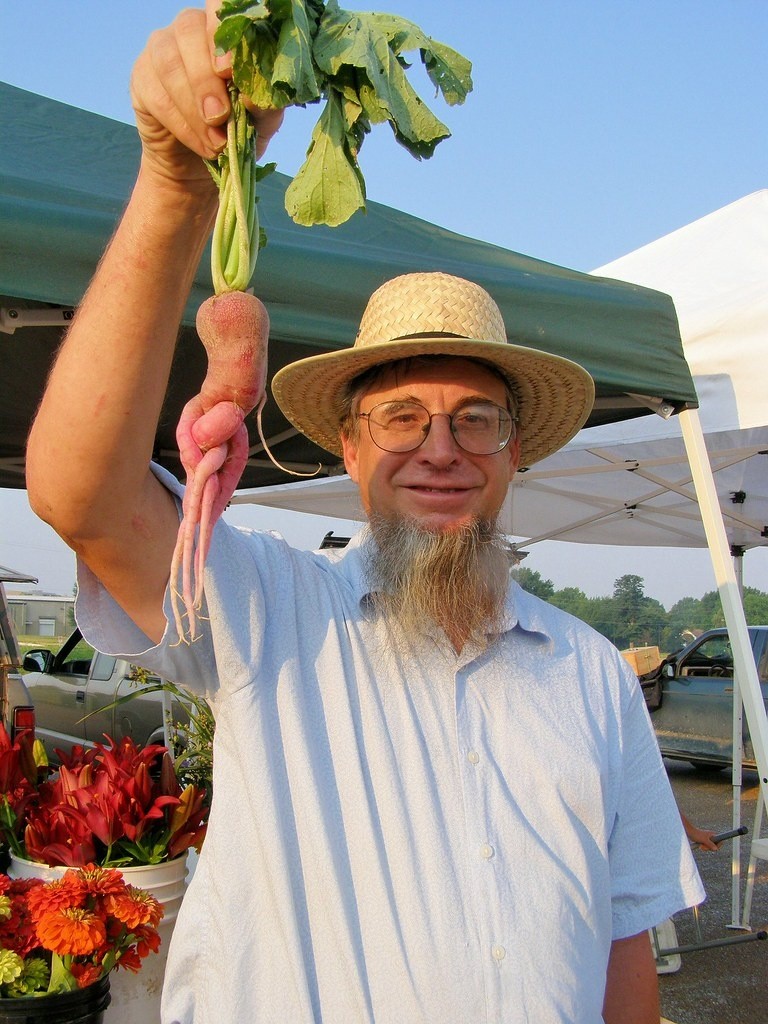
[619,645,661,676]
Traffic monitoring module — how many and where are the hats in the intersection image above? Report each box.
[270,271,596,468]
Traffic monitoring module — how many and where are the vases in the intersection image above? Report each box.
[6,840,189,1024]
[0,973,112,1024]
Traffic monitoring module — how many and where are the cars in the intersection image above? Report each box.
[0,564,38,771]
[630,625,768,775]
[23,623,198,780]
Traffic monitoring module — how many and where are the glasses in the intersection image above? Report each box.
[338,399,520,456]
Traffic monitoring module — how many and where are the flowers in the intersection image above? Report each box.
[0,665,216,1003]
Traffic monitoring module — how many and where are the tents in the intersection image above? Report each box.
[0,82,768,823]
[235,179,768,928]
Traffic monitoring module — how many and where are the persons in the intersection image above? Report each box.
[23,1,706,1024]
